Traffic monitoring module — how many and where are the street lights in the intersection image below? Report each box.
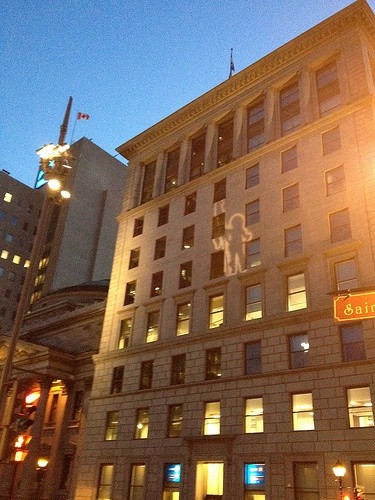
[35,458,49,500]
[331,459,347,500]
[0,141,79,408]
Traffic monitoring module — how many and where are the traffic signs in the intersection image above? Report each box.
[333,291,375,321]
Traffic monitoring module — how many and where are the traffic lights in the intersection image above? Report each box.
[5,388,41,464]
